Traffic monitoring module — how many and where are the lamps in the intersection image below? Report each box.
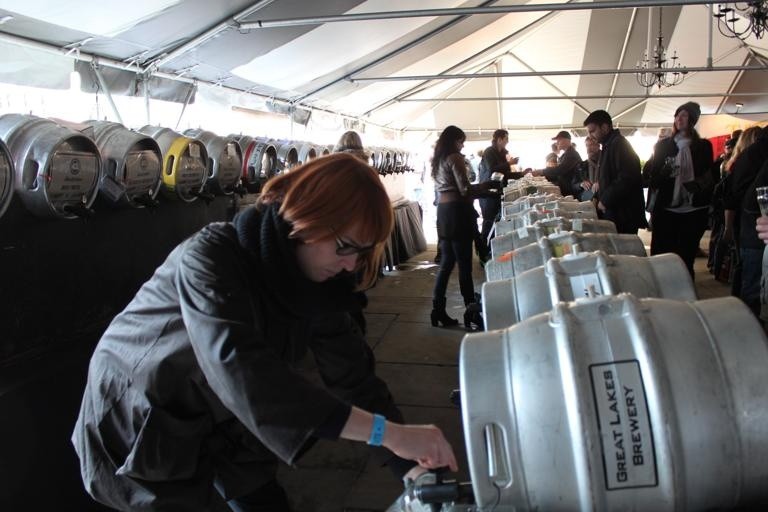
[711,0,768,40]
[635,6,687,88]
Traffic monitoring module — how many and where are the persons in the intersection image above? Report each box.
[479,129,532,268]
[531,131,583,201]
[337,131,368,306]
[545,142,560,167]
[570,135,602,202]
[651,101,714,282]
[642,129,706,257]
[71,155,458,512]
[430,126,484,332]
[433,159,481,265]
[584,110,649,234]
[707,125,768,320]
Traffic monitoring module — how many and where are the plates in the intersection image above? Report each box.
[581,185,595,201]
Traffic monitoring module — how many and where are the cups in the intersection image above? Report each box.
[756,187,768,216]
[385,471,456,512]
[489,171,504,192]
[664,156,679,178]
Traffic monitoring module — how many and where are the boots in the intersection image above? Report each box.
[429,297,459,328]
[463,298,484,329]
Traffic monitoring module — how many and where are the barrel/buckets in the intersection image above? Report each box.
[82,121,163,213]
[363,145,410,173]
[0,139,17,218]
[484,230,648,283]
[503,200,595,220]
[481,252,698,332]
[0,113,102,223]
[310,143,329,157]
[135,124,210,204]
[500,175,561,199]
[179,128,244,195]
[260,138,299,172]
[228,133,276,187]
[458,294,768,512]
[284,141,315,164]
[490,216,617,258]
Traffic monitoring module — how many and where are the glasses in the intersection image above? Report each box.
[326,223,376,257]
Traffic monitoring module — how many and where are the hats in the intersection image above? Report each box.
[678,101,701,121]
[551,131,571,140]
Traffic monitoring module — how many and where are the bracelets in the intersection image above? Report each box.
[367,413,386,447]
